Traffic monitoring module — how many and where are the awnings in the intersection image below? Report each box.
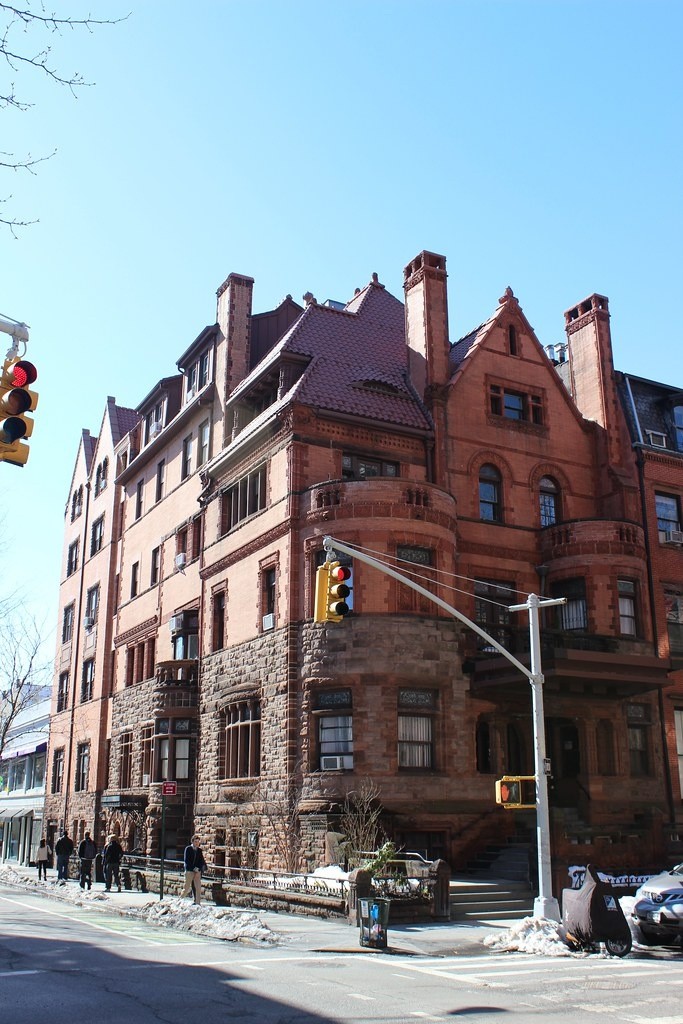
[0,741,47,761]
[0,808,33,823]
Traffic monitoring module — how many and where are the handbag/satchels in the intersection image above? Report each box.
[46,852,53,863]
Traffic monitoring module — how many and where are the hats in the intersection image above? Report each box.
[62,831,68,835]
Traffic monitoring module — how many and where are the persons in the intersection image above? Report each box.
[35,839,52,881]
[55,831,73,882]
[178,835,204,906]
[101,834,123,892]
[77,832,96,890]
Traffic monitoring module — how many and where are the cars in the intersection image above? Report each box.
[631,861,683,948]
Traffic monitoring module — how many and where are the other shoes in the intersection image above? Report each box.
[86,887,91,890]
[116,885,121,892]
[102,890,110,892]
[43,877,47,881]
[38,879,41,880]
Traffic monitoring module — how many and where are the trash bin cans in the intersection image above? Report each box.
[357,897,391,948]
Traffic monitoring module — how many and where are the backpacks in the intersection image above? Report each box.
[83,839,95,859]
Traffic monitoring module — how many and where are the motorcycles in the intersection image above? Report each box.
[561,864,632,957]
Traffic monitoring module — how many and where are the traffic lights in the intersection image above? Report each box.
[315,563,350,623]
[496,780,521,805]
[0,356,38,467]
